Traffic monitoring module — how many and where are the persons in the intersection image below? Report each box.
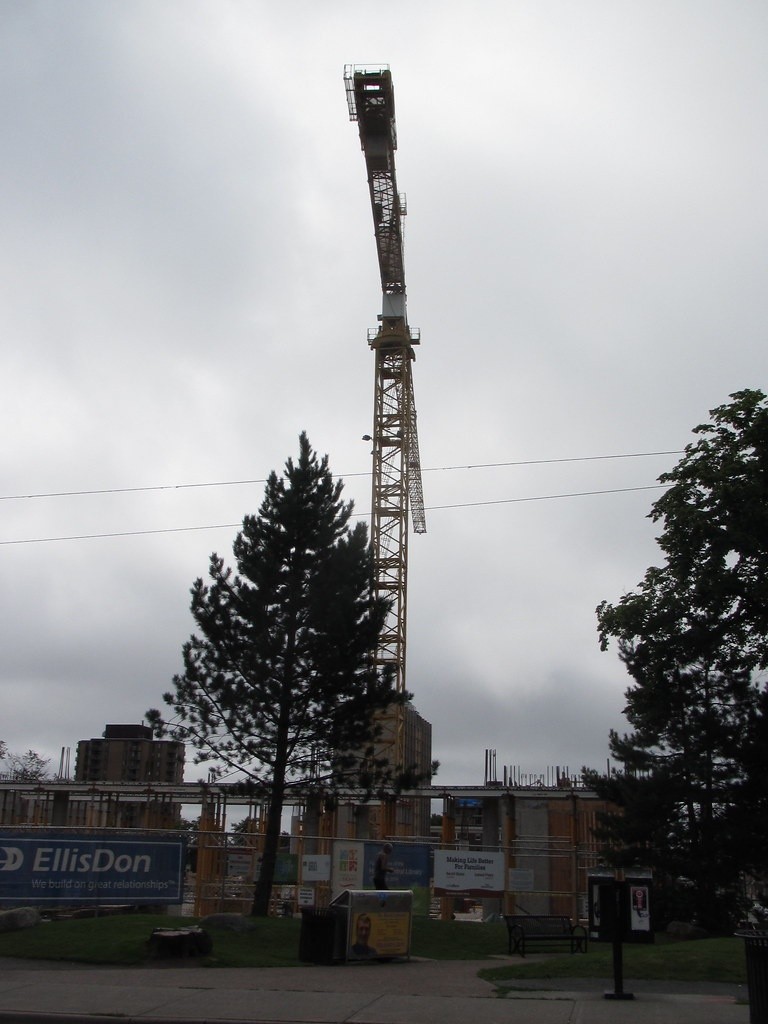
[348,913,377,959]
[373,843,394,890]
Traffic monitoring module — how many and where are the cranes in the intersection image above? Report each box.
[339,60,427,702]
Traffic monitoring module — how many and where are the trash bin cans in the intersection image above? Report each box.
[298,906,347,966]
[733,929,768,1024]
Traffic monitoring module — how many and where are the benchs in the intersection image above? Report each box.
[503,913,589,958]
